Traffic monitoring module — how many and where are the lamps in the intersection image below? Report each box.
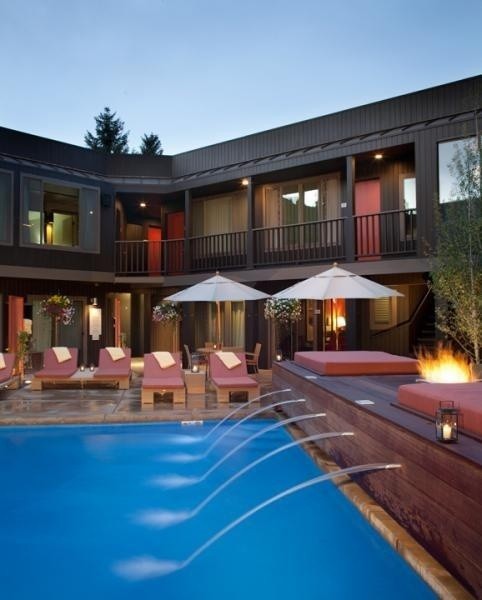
[436,400,462,443]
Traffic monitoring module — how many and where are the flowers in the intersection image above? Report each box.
[153,301,184,326]
[263,298,302,324]
[38,295,75,326]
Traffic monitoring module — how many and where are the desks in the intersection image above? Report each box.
[196,347,217,354]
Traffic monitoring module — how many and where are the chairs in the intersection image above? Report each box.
[94,346,133,389]
[208,352,261,403]
[141,352,186,404]
[245,343,262,373]
[183,343,208,370]
[31,347,80,389]
[0,352,21,393]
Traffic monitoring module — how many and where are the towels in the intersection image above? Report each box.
[0,353,6,369]
[151,351,176,369]
[105,347,125,362]
[52,346,72,363]
[215,351,242,370]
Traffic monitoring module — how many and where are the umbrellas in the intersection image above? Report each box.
[270,262,405,350]
[162,271,271,353]
